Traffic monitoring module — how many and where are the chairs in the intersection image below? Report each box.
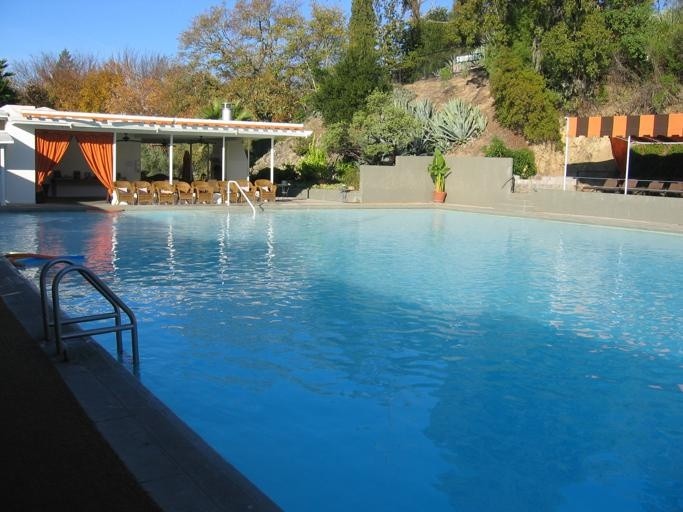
[111,179,278,207]
[580,179,683,196]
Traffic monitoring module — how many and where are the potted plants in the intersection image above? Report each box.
[427,146,451,203]
